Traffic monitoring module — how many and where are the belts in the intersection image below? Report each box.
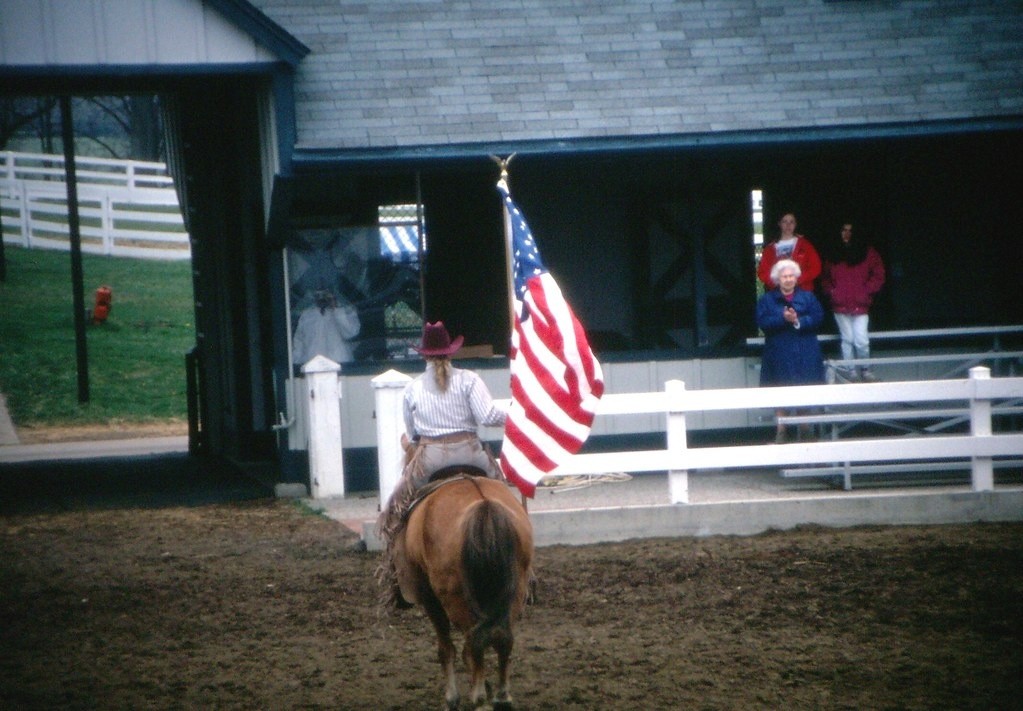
[419,432,477,445]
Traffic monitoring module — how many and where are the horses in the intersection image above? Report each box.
[374,433,534,711]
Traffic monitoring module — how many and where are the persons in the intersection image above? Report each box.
[754,257,825,444]
[813,222,886,379]
[754,210,821,296]
[294,276,362,367]
[380,322,539,608]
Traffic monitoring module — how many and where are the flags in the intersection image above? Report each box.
[480,174,607,503]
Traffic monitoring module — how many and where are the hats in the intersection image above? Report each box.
[407,320,463,356]
